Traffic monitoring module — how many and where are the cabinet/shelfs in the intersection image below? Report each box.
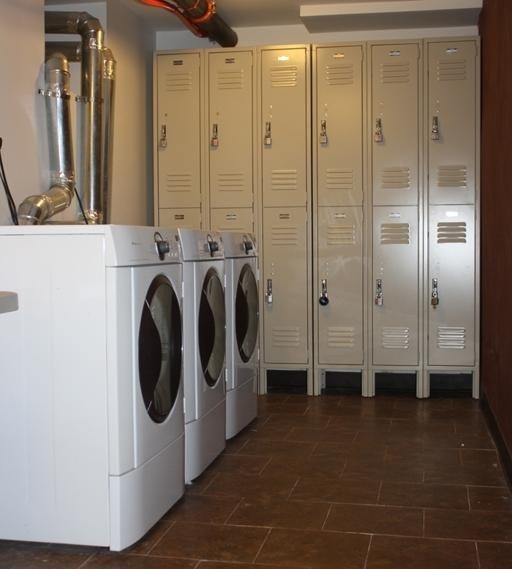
[204,47,260,396]
[153,50,204,231]
[422,35,480,400]
[257,44,314,397]
[311,42,367,397]
[366,39,423,399]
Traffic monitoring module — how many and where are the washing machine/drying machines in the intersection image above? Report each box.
[223,230,260,442]
[1,225,187,554]
[178,227,228,488]
